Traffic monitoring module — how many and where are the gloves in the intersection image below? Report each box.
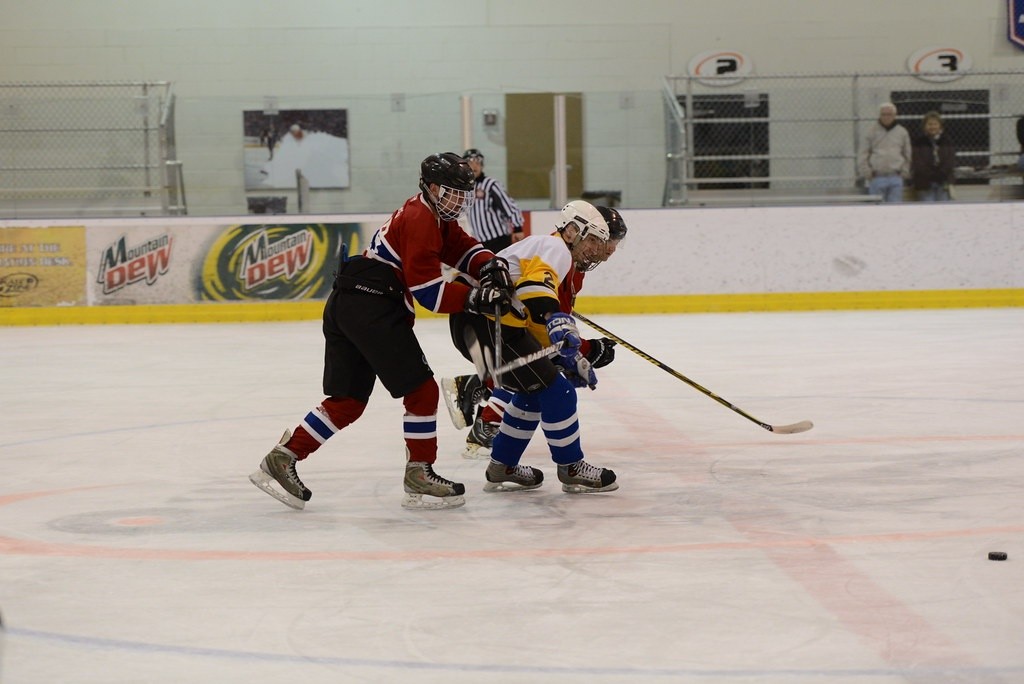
[585,337,617,369]
[545,313,582,357]
[478,256,515,298]
[464,286,512,317]
[559,350,596,387]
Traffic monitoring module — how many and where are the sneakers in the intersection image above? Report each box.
[249,428,312,510]
[557,460,619,493]
[400,445,465,510]
[461,418,500,460]
[442,374,486,430]
[483,460,544,492]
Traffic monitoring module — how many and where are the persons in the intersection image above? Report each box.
[441,205,627,459]
[249,153,515,510]
[462,149,525,253]
[860,103,912,202]
[912,112,956,202]
[465,199,619,493]
[1016,116,1024,179]
[260,122,281,160]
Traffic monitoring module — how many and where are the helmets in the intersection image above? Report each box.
[596,205,627,240]
[462,148,484,167]
[419,152,475,221]
[561,200,610,271]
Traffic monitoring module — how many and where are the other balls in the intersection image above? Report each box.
[988,551,1007,561]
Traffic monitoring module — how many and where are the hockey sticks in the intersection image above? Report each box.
[491,302,569,389]
[563,368,598,392]
[571,307,816,436]
